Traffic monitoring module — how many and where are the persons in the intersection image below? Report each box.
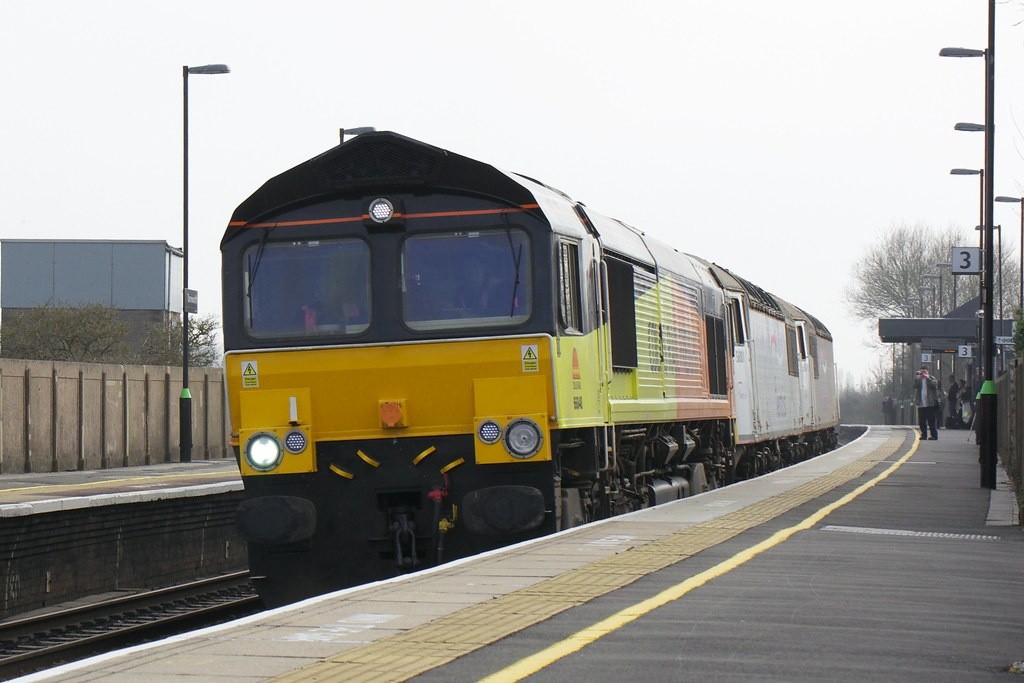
[957,380,965,415]
[883,396,892,424]
[913,366,938,440]
[947,376,957,415]
[936,381,945,426]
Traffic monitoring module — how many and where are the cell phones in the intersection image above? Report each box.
[917,372,923,375]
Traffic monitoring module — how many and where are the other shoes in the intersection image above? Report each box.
[920,436,927,441]
[929,435,938,440]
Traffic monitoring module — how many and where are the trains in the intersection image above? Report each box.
[217,130,838,614]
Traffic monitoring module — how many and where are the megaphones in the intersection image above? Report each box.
[975,310,984,318]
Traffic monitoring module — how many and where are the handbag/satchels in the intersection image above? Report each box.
[945,417,961,430]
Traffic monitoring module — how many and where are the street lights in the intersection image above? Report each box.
[938,262,958,378]
[177,62,234,461]
[974,223,1006,376]
[994,193,1023,324]
[922,272,944,387]
[909,298,924,322]
[338,126,375,145]
[938,45,991,487]
[916,284,936,379]
[902,304,917,426]
